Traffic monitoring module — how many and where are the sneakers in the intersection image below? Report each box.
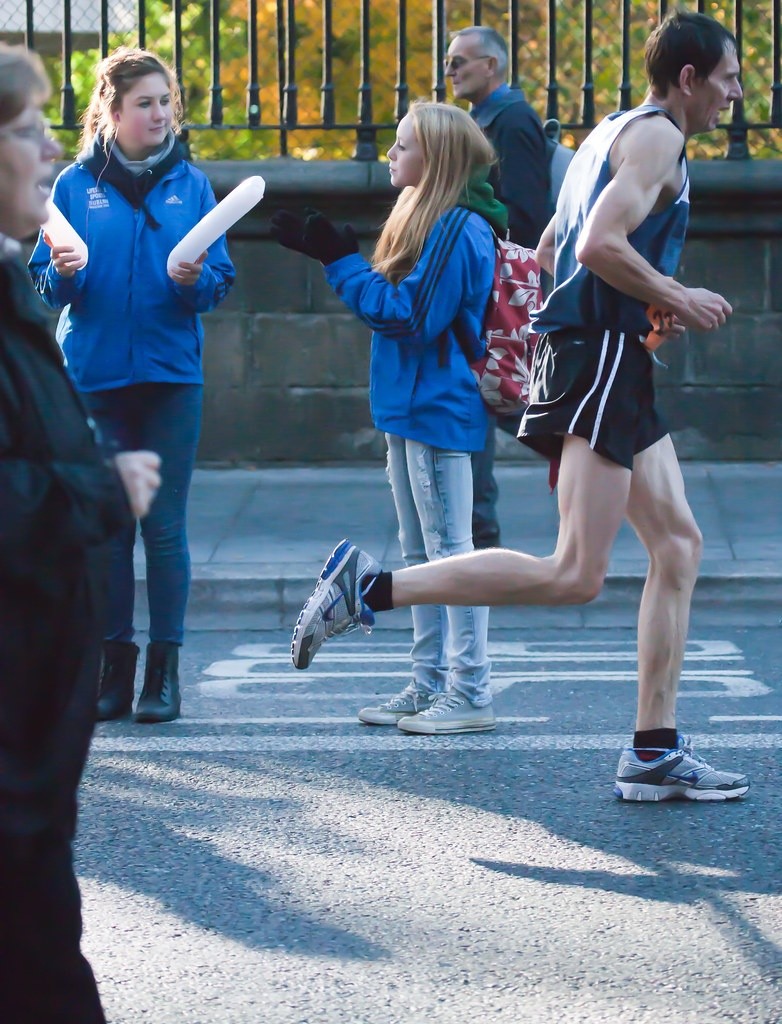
[291,537,383,669]
[358,678,444,724]
[613,735,750,800]
[397,685,495,733]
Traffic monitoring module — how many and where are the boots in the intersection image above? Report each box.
[134,641,182,723]
[93,640,140,722]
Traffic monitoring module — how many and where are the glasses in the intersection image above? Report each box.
[443,55,490,70]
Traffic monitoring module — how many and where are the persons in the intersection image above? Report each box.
[443,26,553,550]
[288,8,753,806]
[267,102,510,735]
[27,46,236,724]
[0,44,162,1024]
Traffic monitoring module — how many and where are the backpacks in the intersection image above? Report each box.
[442,205,544,415]
[542,117,576,226]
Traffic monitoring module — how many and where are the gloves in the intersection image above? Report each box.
[269,208,359,267]
